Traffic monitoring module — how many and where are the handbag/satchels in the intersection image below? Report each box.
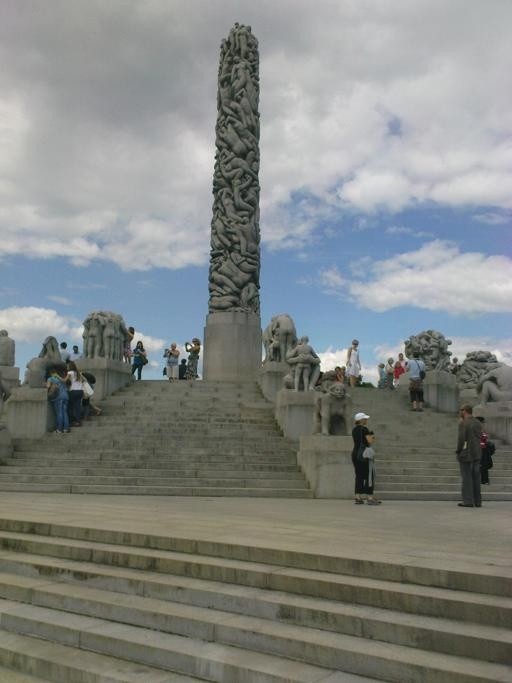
[82,372,96,384]
[82,381,94,400]
[362,447,374,459]
[420,370,425,379]
[48,382,60,400]
[140,355,148,364]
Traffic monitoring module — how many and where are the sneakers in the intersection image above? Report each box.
[354,497,365,504]
[367,497,381,505]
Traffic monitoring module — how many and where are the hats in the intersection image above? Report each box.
[354,412,370,421]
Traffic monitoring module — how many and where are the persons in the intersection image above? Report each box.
[82,311,125,361]
[406,352,425,411]
[164,338,200,379]
[69,346,82,360]
[132,342,146,380]
[404,330,458,373]
[43,362,102,434]
[208,22,261,313]
[475,416,488,484]
[476,362,512,408]
[59,343,70,362]
[456,404,483,507]
[124,327,134,364]
[335,340,407,389]
[262,315,320,393]
[311,382,355,435]
[352,412,382,505]
